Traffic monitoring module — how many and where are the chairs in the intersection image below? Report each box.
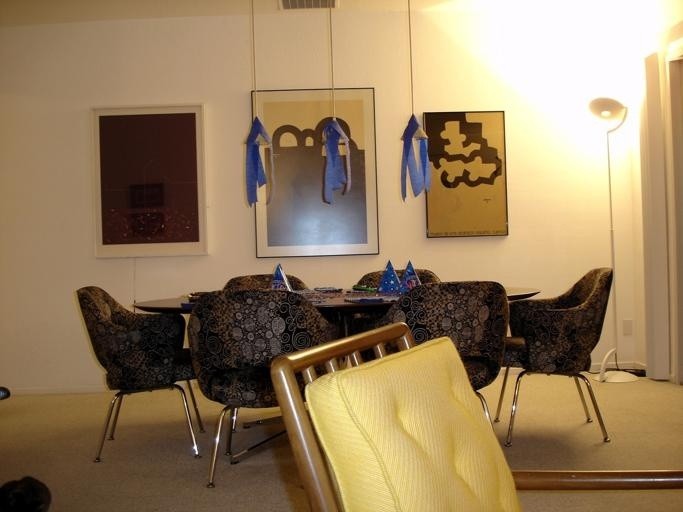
[187,288,331,485]
[494,270,612,450]
[355,270,441,293]
[222,273,307,293]
[71,283,204,464]
[270,320,523,511]
[375,281,511,441]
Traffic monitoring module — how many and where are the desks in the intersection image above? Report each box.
[132,286,541,464]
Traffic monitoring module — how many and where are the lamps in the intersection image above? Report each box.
[239,1,430,207]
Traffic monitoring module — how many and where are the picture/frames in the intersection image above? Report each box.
[421,109,508,239]
[87,100,212,258]
[249,87,382,257]
